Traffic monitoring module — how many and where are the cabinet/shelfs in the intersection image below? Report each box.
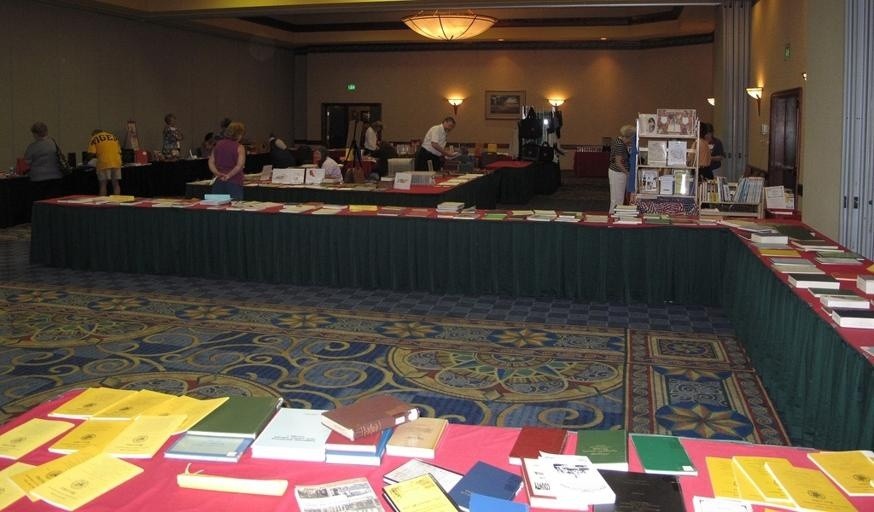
[634,117,701,199]
[697,199,765,223]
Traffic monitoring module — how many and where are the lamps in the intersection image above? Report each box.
[399,6,499,43]
[745,87,764,117]
[544,97,567,112]
[448,97,463,117]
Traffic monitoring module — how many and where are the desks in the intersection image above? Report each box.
[721,218,874,452]
[243,198,725,308]
[485,160,538,206]
[0,363,873,512]
[182,163,388,208]
[0,149,274,232]
[384,168,499,212]
[573,151,610,177]
[28,194,262,282]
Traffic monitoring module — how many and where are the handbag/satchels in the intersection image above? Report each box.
[518,106,554,161]
[53,138,73,176]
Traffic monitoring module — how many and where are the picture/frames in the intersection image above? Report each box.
[484,88,527,121]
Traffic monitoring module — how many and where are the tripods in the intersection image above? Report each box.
[343,140,362,168]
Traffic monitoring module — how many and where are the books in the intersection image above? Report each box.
[0,385,872,511]
[700,176,796,242]
[436,202,697,226]
[71,196,432,218]
[728,209,873,363]
[245,146,485,192]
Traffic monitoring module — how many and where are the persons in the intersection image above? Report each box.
[689,122,714,210]
[605,125,636,209]
[312,146,344,184]
[15,121,65,202]
[206,122,246,200]
[268,132,298,168]
[646,117,656,134]
[82,127,122,196]
[160,113,182,159]
[363,120,383,155]
[704,124,724,178]
[412,117,456,171]
